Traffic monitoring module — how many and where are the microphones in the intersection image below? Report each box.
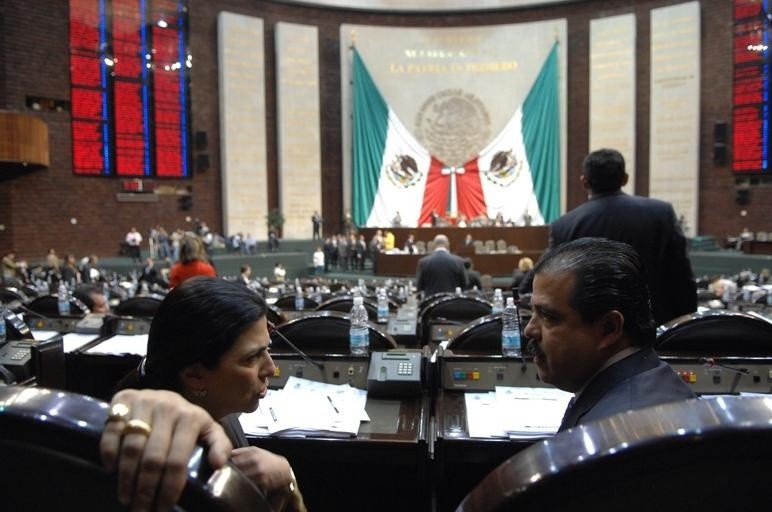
[264,320,323,369]
[511,285,528,372]
[700,356,750,375]
[16,304,56,325]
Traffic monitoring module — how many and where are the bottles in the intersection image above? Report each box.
[295,288,304,310]
[348,298,369,356]
[0,299,8,345]
[375,289,388,322]
[501,296,523,358]
[492,288,503,312]
[58,285,70,315]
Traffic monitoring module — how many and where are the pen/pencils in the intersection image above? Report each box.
[327,396,339,413]
[270,408,277,421]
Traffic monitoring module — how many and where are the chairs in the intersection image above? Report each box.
[415,238,508,251]
[454,394,772,512]
[1,271,771,454]
[1,384,267,509]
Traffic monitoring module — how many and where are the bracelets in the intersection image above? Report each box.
[271,467,299,495]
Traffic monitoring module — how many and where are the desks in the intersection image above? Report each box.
[740,240,771,255]
[375,252,542,277]
[359,225,552,257]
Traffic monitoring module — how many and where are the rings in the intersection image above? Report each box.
[98,402,131,422]
[122,420,152,436]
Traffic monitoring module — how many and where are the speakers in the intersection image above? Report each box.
[197,154,209,169]
[195,132,208,150]
[713,121,727,140]
[713,144,726,164]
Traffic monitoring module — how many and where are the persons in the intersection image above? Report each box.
[125,217,281,259]
[710,269,772,315]
[312,230,417,272]
[389,209,533,227]
[512,257,534,290]
[544,146,697,335]
[310,212,322,240]
[415,234,482,300]
[523,235,701,444]
[238,262,287,285]
[727,227,754,252]
[96,274,307,511]
[1,245,110,313]
